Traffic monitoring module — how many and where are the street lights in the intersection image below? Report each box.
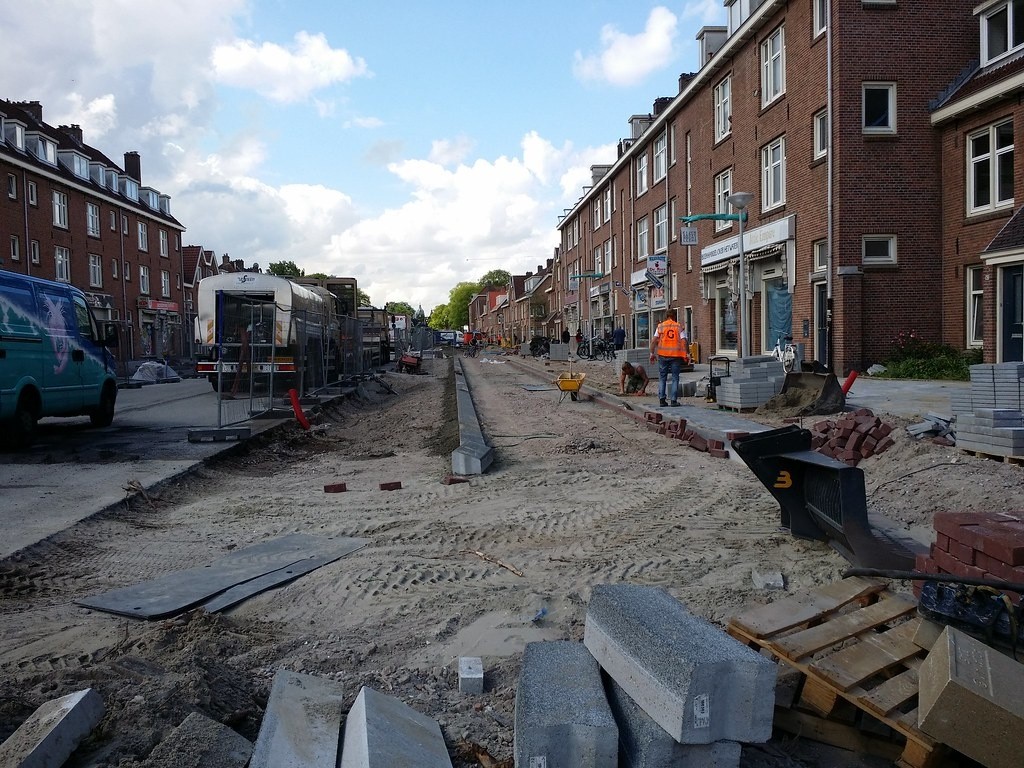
[724,191,756,358]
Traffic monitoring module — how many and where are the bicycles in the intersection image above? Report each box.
[576,332,615,363]
[462,345,479,359]
[768,326,797,375]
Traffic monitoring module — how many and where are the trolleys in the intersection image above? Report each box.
[551,372,587,403]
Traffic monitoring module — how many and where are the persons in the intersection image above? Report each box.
[561,327,571,354]
[613,325,626,350]
[649,308,691,406]
[619,361,649,396]
[576,329,584,348]
[513,334,518,345]
[497,334,501,345]
[469,336,477,356]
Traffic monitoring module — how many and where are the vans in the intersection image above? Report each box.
[0,269,121,448]
[441,331,465,345]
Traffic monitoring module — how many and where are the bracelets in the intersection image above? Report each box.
[650,352,654,354]
[687,353,690,354]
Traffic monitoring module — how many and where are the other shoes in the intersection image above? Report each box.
[671,400,680,406]
[660,399,668,407]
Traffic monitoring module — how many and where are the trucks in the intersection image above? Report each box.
[191,271,343,392]
[358,306,396,366]
[387,315,412,352]
